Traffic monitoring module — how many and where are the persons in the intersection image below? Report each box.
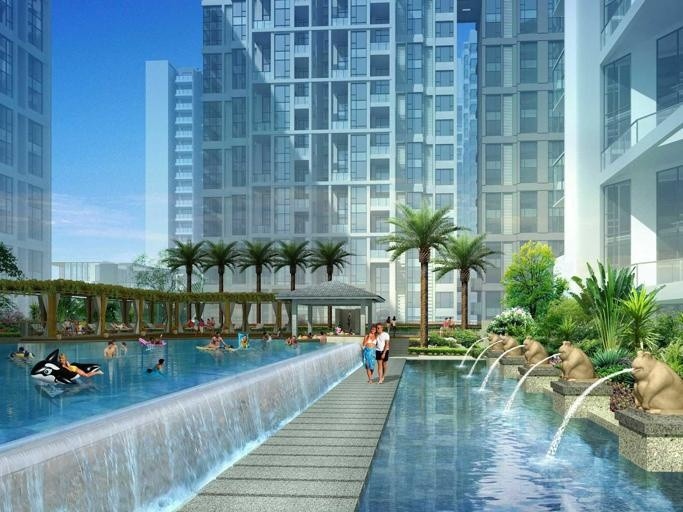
[145,359,165,376]
[335,311,353,336]
[208,331,235,352]
[59,353,104,378]
[65,319,71,337]
[206,317,216,329]
[287,336,298,345]
[319,331,326,344]
[74,319,78,335]
[241,335,248,349]
[104,340,127,358]
[262,332,272,341]
[150,334,163,344]
[307,333,313,340]
[188,317,204,337]
[443,317,455,328]
[360,316,397,385]
[10,347,36,360]
